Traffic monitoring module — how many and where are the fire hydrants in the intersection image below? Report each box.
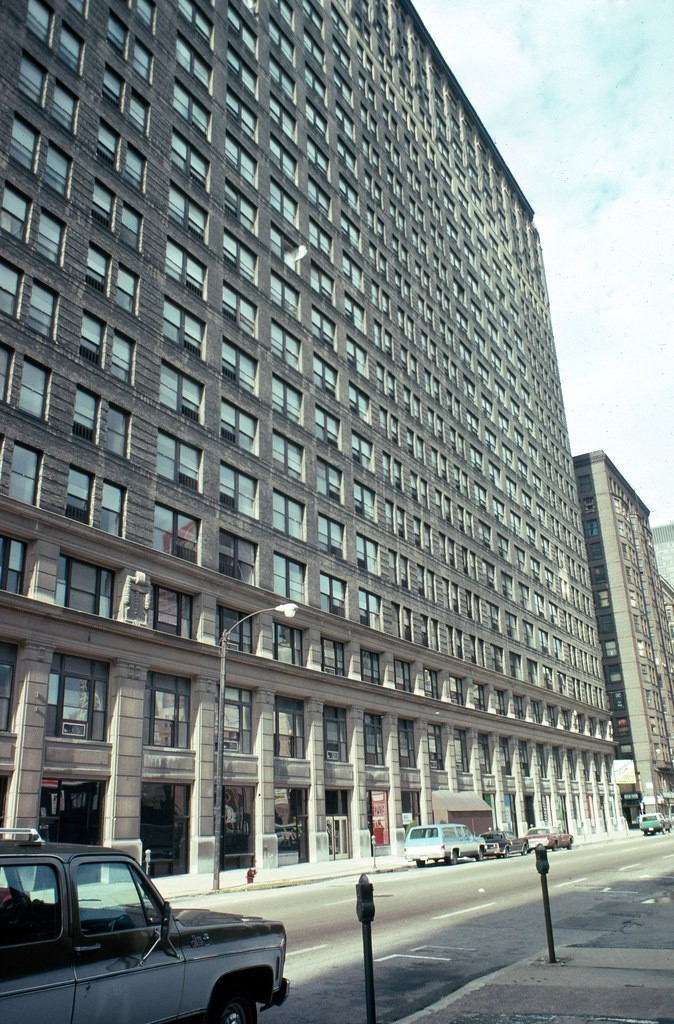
[246,868,256,884]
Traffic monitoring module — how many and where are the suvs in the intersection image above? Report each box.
[639,813,669,836]
[0,827,290,1024]
[404,823,488,869]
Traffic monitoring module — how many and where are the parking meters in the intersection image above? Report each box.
[534,843,558,964]
[355,873,377,1024]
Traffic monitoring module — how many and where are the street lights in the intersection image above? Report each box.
[212,603,298,888]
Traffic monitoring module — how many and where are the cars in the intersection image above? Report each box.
[524,827,574,852]
[479,831,530,859]
